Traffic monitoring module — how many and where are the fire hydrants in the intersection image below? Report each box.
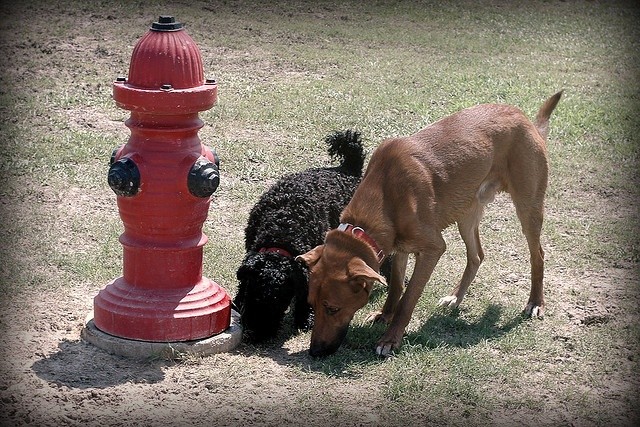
[93,13,233,344]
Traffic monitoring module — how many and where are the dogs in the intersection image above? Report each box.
[294,88,567,357]
[233,128,369,342]
